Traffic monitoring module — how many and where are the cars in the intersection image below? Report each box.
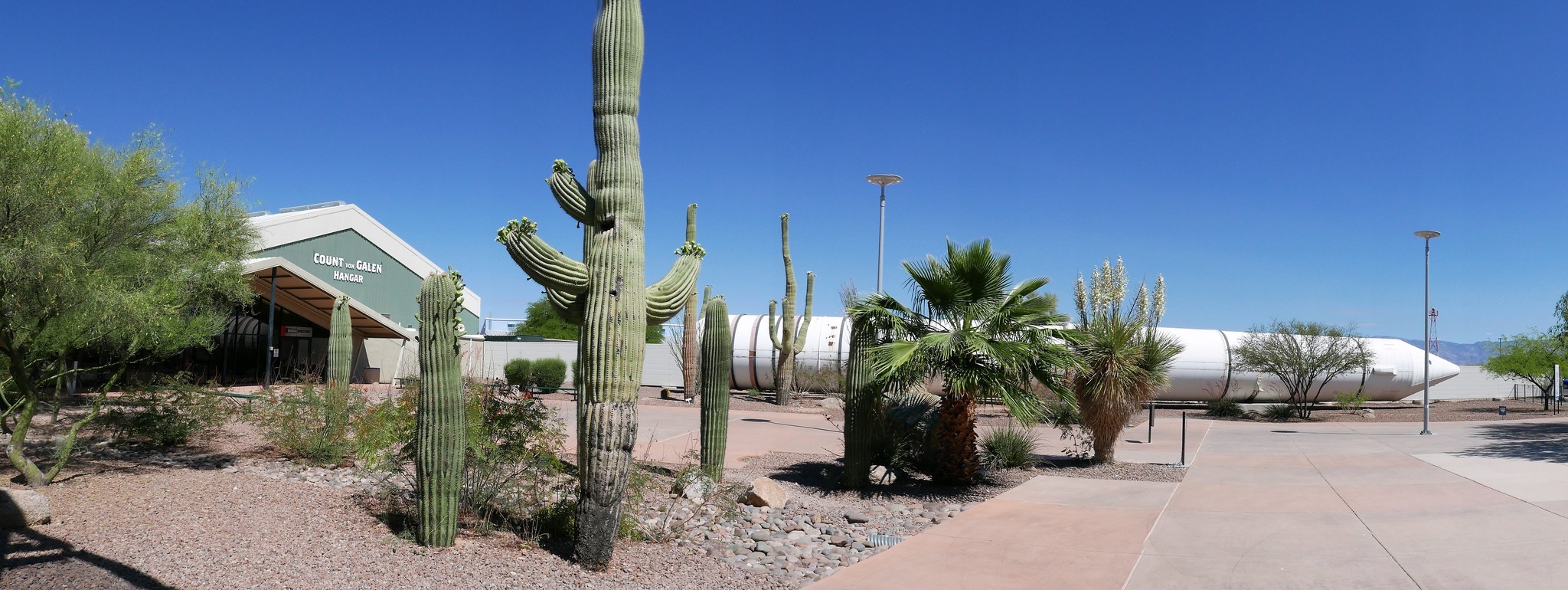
[1563,380,1568,390]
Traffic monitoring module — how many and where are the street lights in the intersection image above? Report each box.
[1498,338,1503,356]
[1414,230,1442,435]
[864,173,904,344]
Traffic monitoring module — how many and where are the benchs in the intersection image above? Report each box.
[394,378,420,389]
[481,384,502,393]
[525,386,577,401]
